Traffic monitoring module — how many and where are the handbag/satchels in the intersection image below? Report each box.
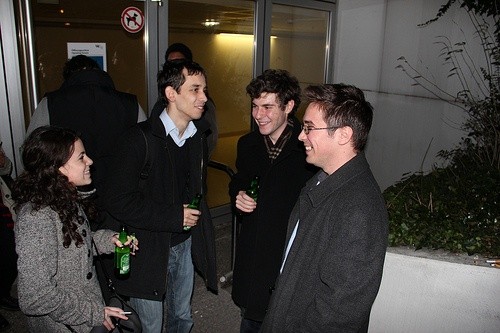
[88,291,144,333]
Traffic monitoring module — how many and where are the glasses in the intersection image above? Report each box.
[300,123,342,133]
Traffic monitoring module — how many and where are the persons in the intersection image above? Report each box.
[257,84,389,333]
[104,57,219,333]
[11,125,143,333]
[24,54,148,203]
[229,67,319,333]
[0,141,22,333]
[151,41,218,151]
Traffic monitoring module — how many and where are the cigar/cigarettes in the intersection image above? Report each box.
[119,311,131,314]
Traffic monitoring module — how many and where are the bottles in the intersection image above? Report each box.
[246,176,260,202]
[182,194,201,230]
[114,221,131,279]
